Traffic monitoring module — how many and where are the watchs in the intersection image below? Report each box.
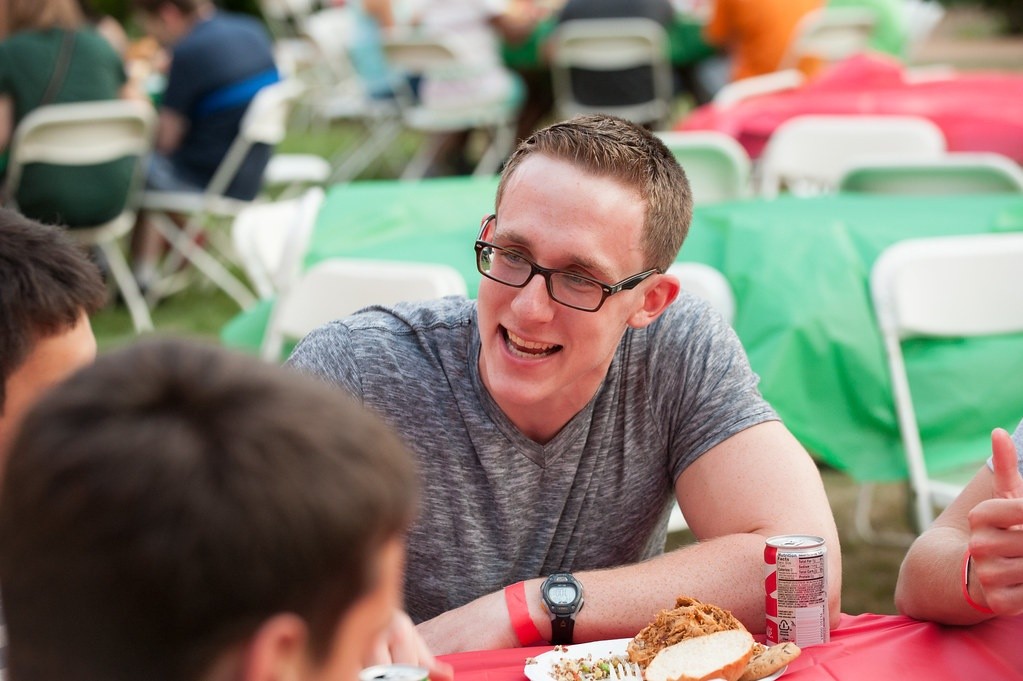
[540,573,585,645]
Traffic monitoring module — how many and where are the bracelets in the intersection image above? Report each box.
[505,582,542,646]
[962,551,991,615]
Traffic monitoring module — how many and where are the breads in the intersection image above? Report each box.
[644,629,755,681]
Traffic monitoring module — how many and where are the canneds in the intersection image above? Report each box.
[763,535,830,648]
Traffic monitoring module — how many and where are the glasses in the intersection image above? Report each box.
[475,214,660,312]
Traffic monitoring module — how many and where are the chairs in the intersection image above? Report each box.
[656,133,753,204]
[869,231,1022,535]
[758,115,1023,205]
[546,17,672,133]
[132,83,302,314]
[279,9,523,179]
[1,95,160,333]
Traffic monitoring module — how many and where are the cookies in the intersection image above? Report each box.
[738,641,801,681]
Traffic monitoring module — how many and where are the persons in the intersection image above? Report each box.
[694,0,824,106]
[0,210,101,471]
[114,0,278,310]
[0,342,419,680]
[355,0,547,173]
[558,0,676,108]
[0,0,132,230]
[276,116,841,681]
[893,419,1023,627]
